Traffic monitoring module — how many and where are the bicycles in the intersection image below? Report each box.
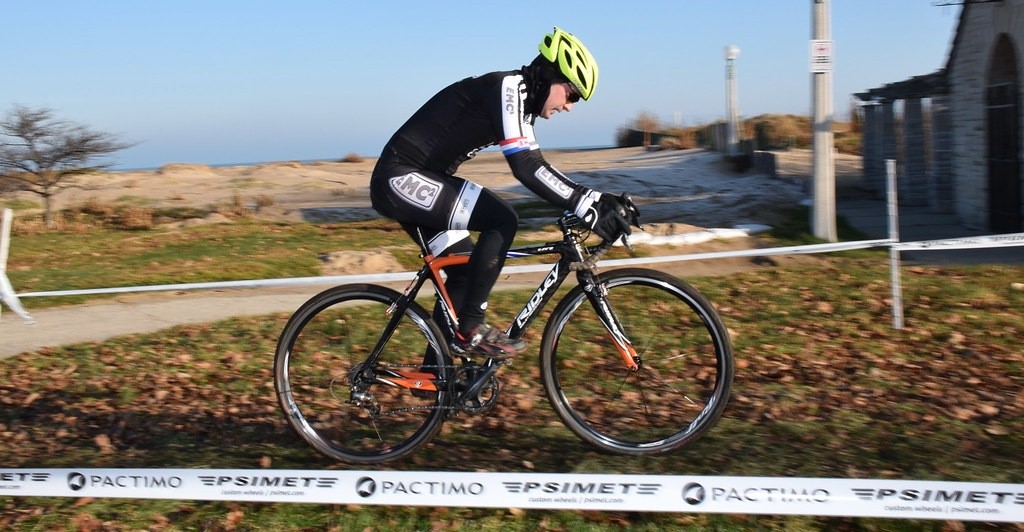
[273,192,734,466]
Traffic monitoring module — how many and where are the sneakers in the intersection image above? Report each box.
[410,374,488,399]
[450,324,528,359]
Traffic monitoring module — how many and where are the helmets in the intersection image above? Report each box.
[539,26,598,103]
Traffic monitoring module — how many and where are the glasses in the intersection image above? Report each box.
[567,93,581,103]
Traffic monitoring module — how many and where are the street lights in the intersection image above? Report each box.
[724,45,742,155]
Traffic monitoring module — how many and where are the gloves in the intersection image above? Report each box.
[568,184,641,242]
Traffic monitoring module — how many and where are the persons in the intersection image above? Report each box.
[369,25,632,399]
